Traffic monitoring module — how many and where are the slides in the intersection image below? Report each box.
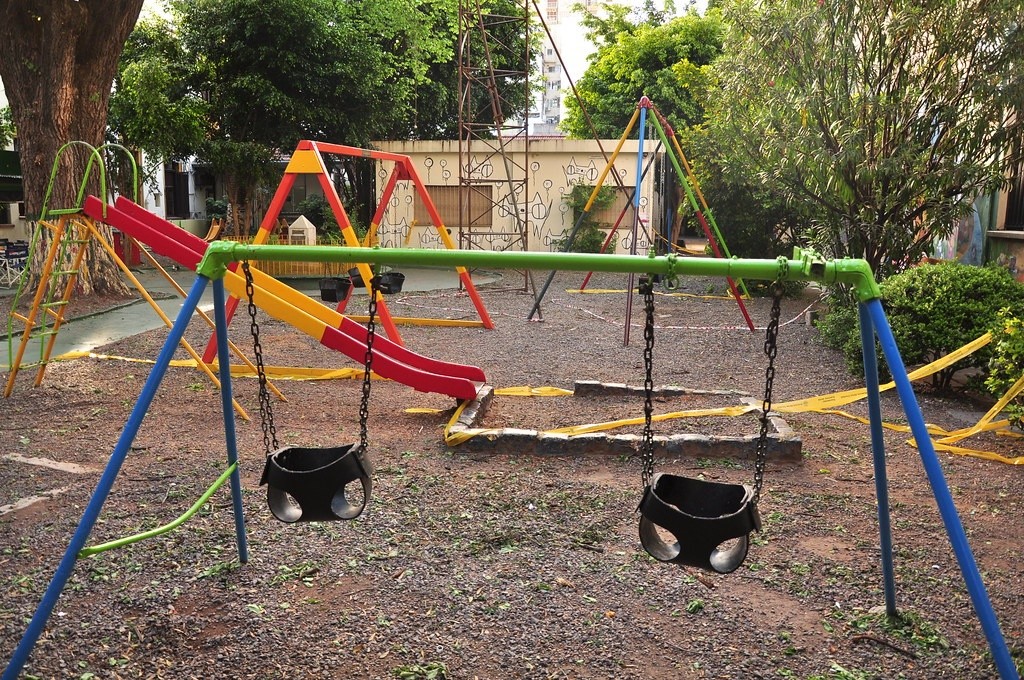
[83,195,487,400]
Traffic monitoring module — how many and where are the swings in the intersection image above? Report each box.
[634,284,784,574]
[638,120,670,293]
[347,157,377,289]
[241,262,383,523]
[319,155,352,302]
[378,162,405,294]
